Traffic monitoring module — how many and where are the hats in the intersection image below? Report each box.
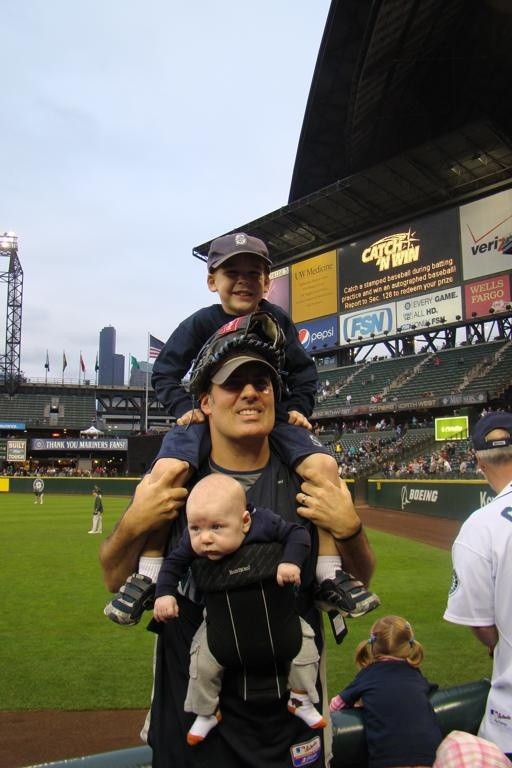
[472,411,512,451]
[209,347,279,386]
[207,232,273,269]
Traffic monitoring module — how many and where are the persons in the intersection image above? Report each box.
[153,472,328,748]
[102,232,381,627]
[310,356,511,479]
[0,454,120,477]
[99,312,377,767]
[87,485,103,534]
[328,616,443,767]
[32,473,45,505]
[441,411,511,764]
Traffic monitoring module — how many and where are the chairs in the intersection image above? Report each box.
[311,338,512,412]
[314,417,512,480]
[1,392,100,434]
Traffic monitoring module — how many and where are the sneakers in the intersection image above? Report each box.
[103,572,156,627]
[311,570,381,619]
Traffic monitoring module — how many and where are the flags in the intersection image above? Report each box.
[79,354,86,374]
[44,354,51,372]
[94,356,101,371]
[150,334,166,357]
[130,356,141,370]
[62,354,68,371]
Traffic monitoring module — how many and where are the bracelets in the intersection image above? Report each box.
[332,523,364,542]
